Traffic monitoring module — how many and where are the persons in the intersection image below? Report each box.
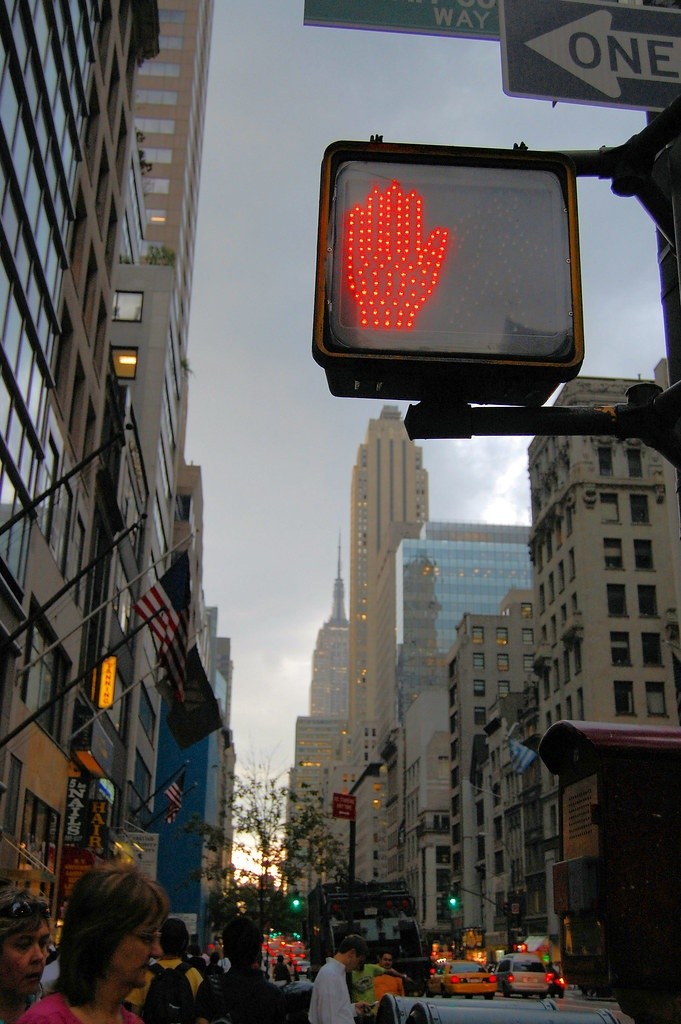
[0,853,291,1024]
[293,965,300,982]
[307,967,311,980]
[547,963,561,998]
[272,954,292,983]
[308,934,416,1024]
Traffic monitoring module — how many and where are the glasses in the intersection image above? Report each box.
[129,928,155,944]
[0,901,51,919]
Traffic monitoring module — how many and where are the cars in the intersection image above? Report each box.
[426,953,498,1000]
[262,935,310,975]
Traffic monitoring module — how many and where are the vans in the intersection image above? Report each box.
[495,953,549,999]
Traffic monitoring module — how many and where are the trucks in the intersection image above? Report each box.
[306,877,430,997]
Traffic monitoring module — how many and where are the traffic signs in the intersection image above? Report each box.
[499,1,681,112]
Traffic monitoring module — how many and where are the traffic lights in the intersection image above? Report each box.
[293,889,301,910]
[449,889,458,908]
[311,139,586,406]
[293,931,301,939]
[269,931,282,937]
[511,942,526,953]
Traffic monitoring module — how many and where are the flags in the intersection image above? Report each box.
[164,768,186,824]
[155,608,189,703]
[133,550,192,659]
[509,737,537,776]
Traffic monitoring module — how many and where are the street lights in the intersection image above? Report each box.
[478,832,514,892]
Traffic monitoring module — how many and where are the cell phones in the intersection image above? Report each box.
[365,1001,378,1009]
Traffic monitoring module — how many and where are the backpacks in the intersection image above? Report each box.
[141,962,197,1023]
